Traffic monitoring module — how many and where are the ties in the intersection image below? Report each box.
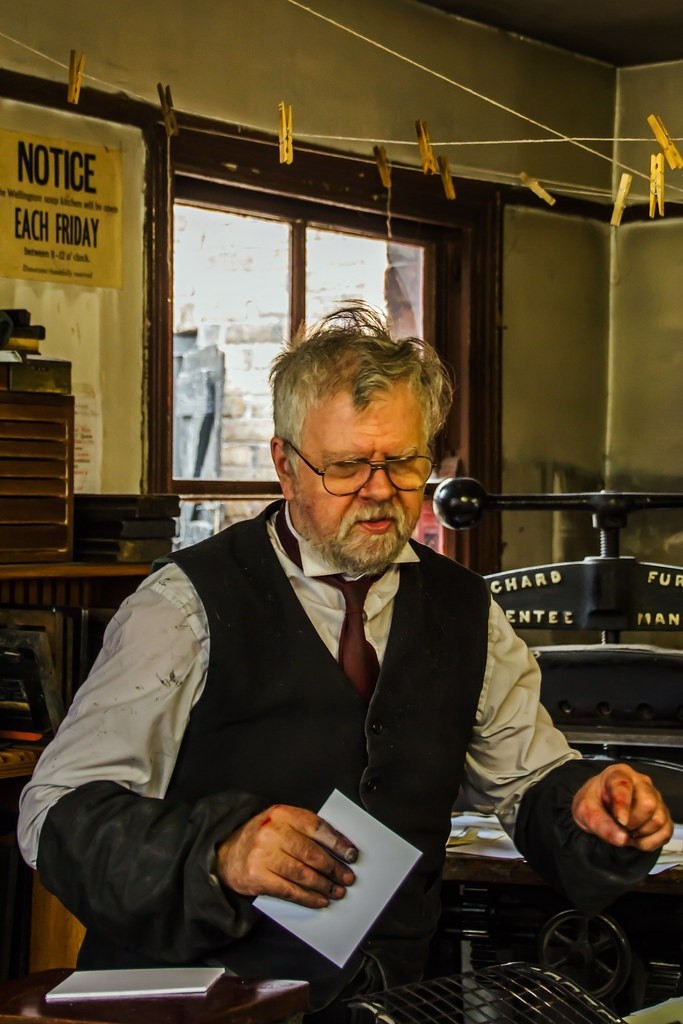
[276,503,392,701]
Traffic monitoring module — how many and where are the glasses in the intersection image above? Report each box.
[284,439,435,495]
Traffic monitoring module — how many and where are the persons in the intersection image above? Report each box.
[17,308,674,1024]
